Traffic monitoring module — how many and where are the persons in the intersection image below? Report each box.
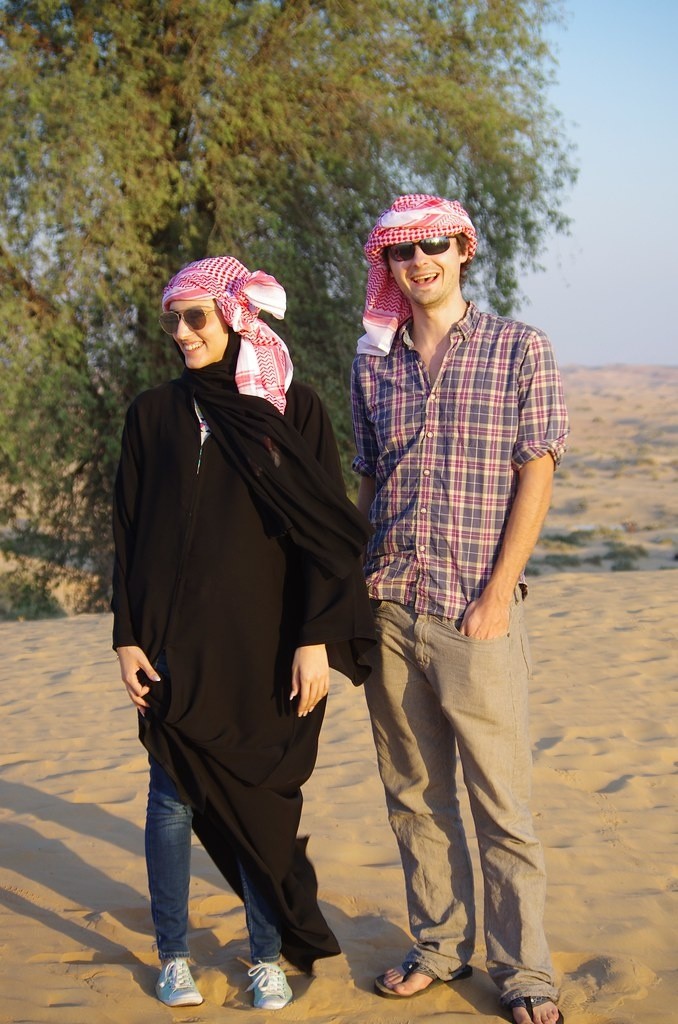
[108,256,380,1016]
[350,192,574,1024]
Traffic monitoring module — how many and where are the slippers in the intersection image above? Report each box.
[374,962,472,999]
[509,997,565,1024]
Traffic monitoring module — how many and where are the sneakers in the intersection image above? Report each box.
[155,957,203,1006]
[245,959,293,1009]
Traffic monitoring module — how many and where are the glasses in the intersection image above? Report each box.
[158,308,219,334]
[388,236,458,261]
